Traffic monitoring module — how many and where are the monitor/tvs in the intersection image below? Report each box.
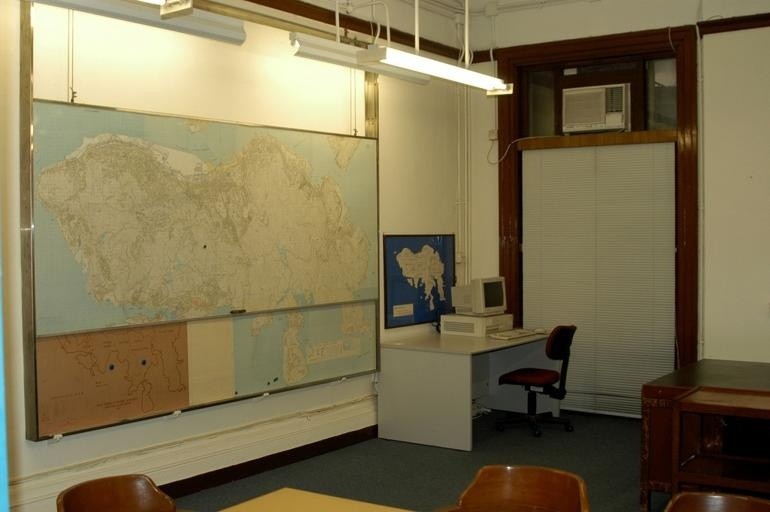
[451,277,508,316]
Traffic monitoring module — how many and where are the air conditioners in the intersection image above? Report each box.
[561,82,633,136]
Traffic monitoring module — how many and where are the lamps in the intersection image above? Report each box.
[38,1,249,48]
[357,44,515,96]
[287,30,431,86]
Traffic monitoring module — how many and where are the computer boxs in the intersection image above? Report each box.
[440,313,514,338]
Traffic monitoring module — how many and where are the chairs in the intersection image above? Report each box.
[662,489,770,512]
[497,323,577,438]
[56,473,177,512]
[455,464,591,512]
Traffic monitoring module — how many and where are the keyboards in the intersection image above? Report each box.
[488,327,536,340]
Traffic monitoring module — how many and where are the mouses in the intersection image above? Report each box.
[533,327,546,334]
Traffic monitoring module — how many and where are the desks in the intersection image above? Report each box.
[378,326,562,452]
[638,358,770,512]
[216,486,415,512]
